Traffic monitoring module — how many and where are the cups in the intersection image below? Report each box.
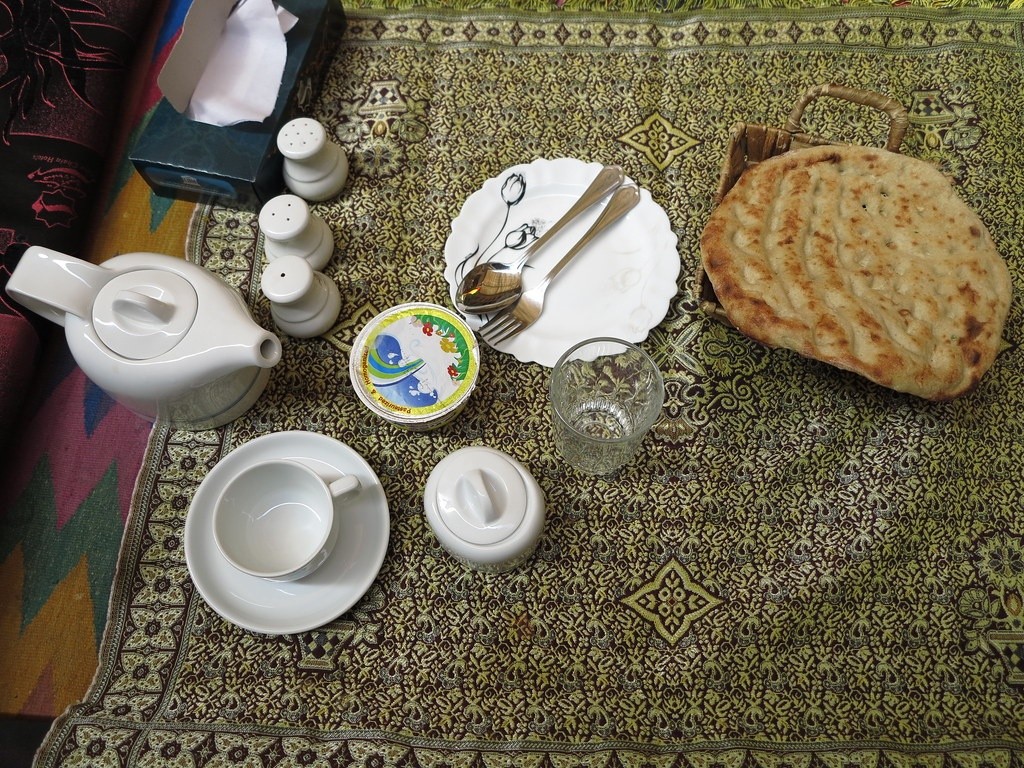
[550,337,665,475]
[212,459,362,582]
[424,448,546,572]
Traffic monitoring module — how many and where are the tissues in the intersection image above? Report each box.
[125,0,349,215]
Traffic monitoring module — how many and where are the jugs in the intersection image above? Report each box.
[5,245,282,430]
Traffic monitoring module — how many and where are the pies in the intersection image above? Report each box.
[699,145,1013,399]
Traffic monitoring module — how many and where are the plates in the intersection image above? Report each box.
[443,158,680,368]
[184,430,390,635]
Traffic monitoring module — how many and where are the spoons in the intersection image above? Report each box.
[456,164,624,314]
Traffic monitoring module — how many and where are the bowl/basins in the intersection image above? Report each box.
[349,302,480,431]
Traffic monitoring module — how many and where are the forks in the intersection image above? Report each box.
[478,184,640,346]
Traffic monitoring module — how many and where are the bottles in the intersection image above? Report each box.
[277,117,349,201]
[261,255,341,339]
[258,194,333,271]
